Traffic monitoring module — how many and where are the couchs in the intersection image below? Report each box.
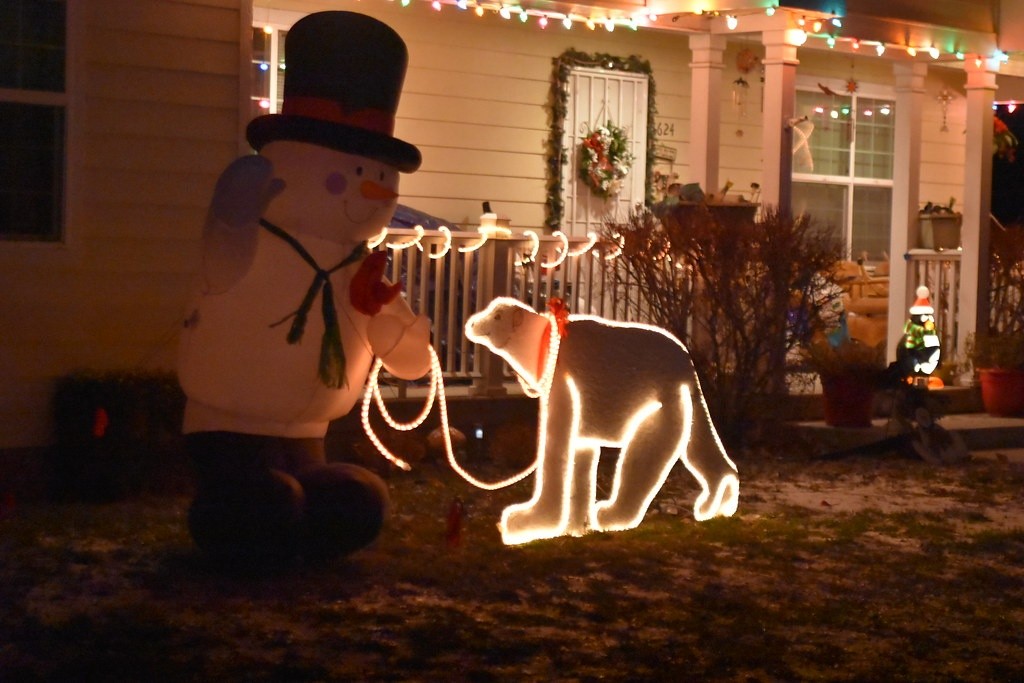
[791,260,889,359]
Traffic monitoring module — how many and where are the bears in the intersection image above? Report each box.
[461,296,740,545]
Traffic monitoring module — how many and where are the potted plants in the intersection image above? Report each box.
[963,325,1024,417]
[917,197,963,251]
[787,334,887,429]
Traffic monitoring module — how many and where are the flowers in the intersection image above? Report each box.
[579,121,636,197]
